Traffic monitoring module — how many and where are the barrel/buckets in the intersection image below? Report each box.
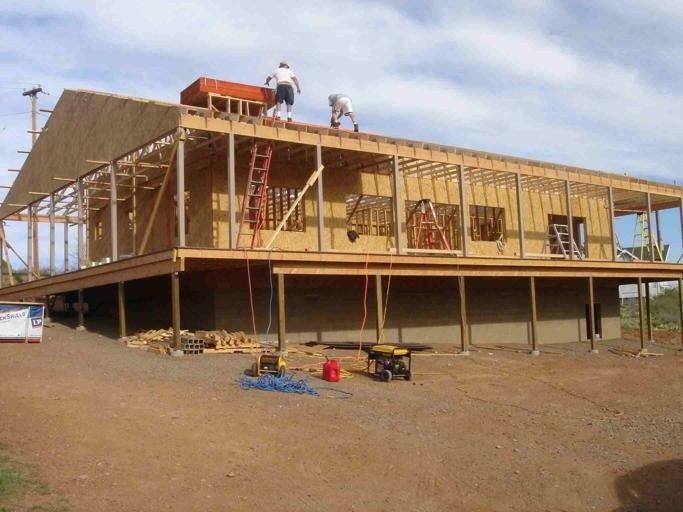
[323,359,341,382]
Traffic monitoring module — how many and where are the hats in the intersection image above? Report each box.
[279,61,289,68]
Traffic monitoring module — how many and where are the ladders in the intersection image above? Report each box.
[631,211,664,261]
[542,223,583,260]
[237,144,273,249]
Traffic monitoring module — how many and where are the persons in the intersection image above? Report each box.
[263,62,301,123]
[328,94,358,132]
